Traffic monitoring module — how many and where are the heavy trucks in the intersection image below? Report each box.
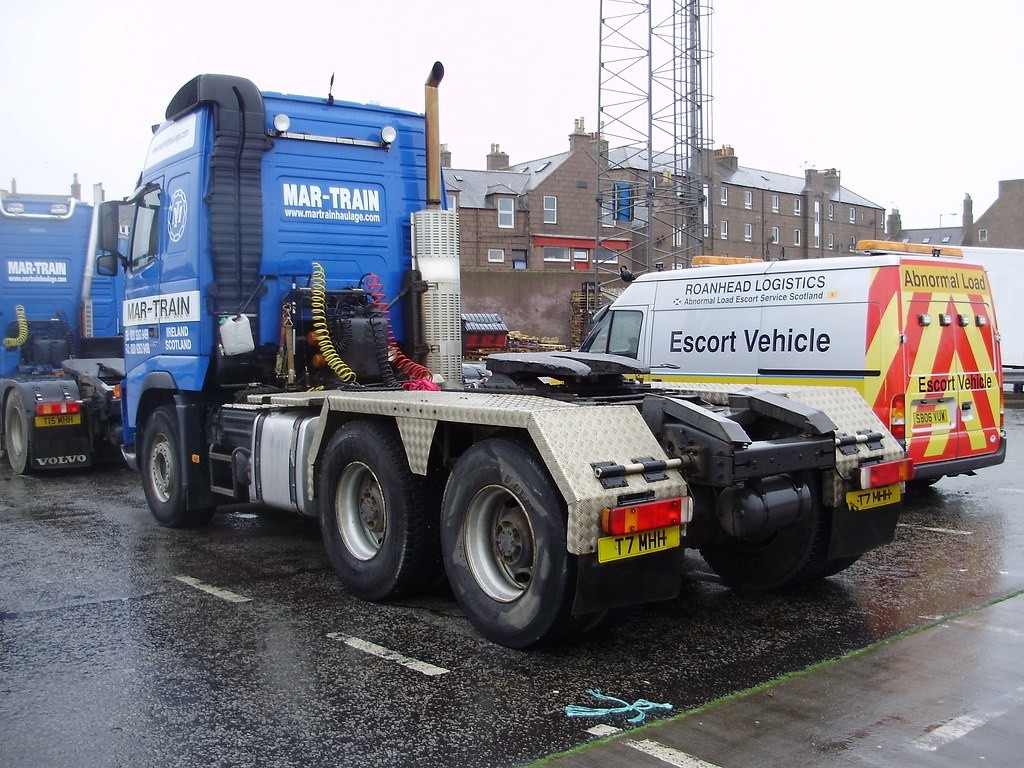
[97,60,914,648]
[0,173,129,475]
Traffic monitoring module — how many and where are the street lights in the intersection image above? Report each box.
[939,213,957,244]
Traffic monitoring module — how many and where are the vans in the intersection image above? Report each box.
[579,240,1007,488]
